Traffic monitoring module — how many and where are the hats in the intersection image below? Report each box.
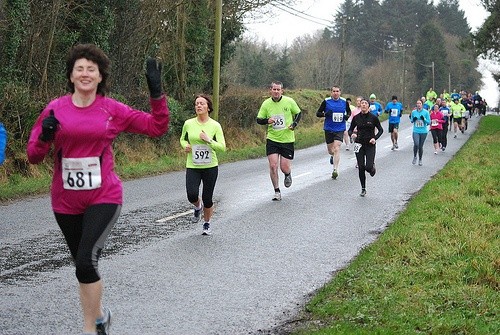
[370,94,376,99]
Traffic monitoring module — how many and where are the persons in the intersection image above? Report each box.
[420,87,487,154]
[410,100,430,166]
[384,95,403,150]
[348,98,384,196]
[316,85,352,179]
[28,43,169,335]
[352,96,370,168]
[344,98,355,150]
[180,95,225,236]
[257,81,301,200]
[369,94,382,117]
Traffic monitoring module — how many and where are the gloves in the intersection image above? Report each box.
[144,58,162,98]
[325,110,333,117]
[400,113,401,118]
[385,110,388,114]
[343,115,348,120]
[420,116,424,120]
[413,117,417,121]
[40,109,61,141]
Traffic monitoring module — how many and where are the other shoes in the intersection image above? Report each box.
[412,157,417,164]
[439,143,445,151]
[419,158,423,164]
[461,127,464,133]
[454,134,457,138]
[434,151,437,154]
[356,165,358,168]
[392,143,398,150]
[345,145,350,150]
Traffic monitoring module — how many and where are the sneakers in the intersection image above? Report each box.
[273,192,282,200]
[370,164,375,177]
[332,170,338,179]
[95,307,112,335]
[361,189,366,196]
[284,173,292,187]
[330,156,334,164]
[193,205,203,223]
[201,222,211,235]
